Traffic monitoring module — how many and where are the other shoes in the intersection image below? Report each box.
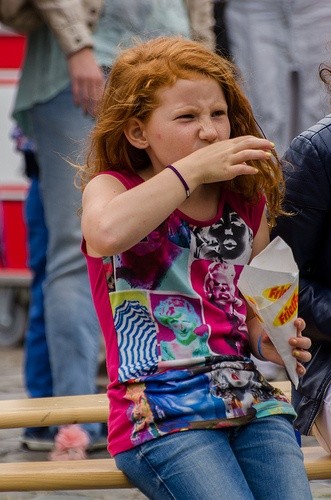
[19,427,107,450]
[48,423,88,461]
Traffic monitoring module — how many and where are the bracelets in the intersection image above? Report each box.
[258,332,266,361]
[167,164,190,199]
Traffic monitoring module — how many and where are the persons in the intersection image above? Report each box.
[269,63,331,454]
[0,0,330,451]
[81,38,312,500]
